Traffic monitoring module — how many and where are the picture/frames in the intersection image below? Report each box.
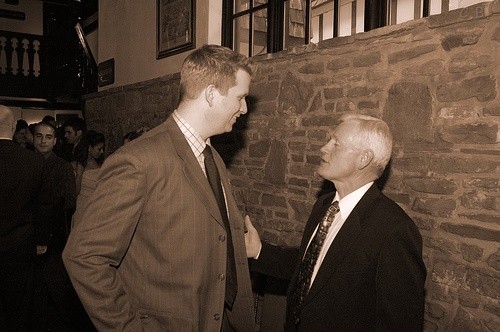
[156,0,196,60]
[56,113,79,128]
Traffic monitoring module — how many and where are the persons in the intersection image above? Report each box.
[0,105,149,332]
[244,113,427,332]
[62,45,256,332]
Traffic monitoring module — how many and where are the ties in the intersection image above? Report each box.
[203,144,239,314]
[285,201,341,332]
